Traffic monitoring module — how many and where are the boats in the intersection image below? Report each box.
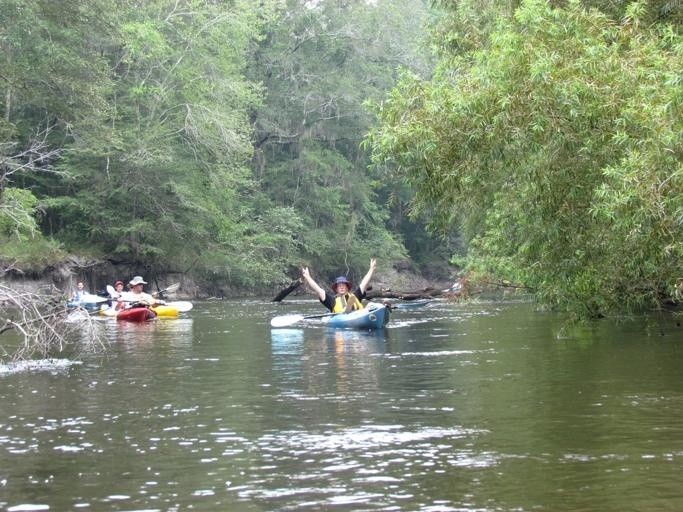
[320,302,390,330]
[67,294,178,322]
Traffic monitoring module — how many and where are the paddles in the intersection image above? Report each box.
[107,284,115,297]
[304,300,430,320]
[82,294,139,304]
[151,282,180,296]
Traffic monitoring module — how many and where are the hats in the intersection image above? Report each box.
[126,276,147,289]
[113,281,123,288]
[331,277,352,292]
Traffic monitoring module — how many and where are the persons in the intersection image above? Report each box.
[452,278,461,291]
[71,282,89,302]
[105,276,159,311]
[302,258,377,314]
